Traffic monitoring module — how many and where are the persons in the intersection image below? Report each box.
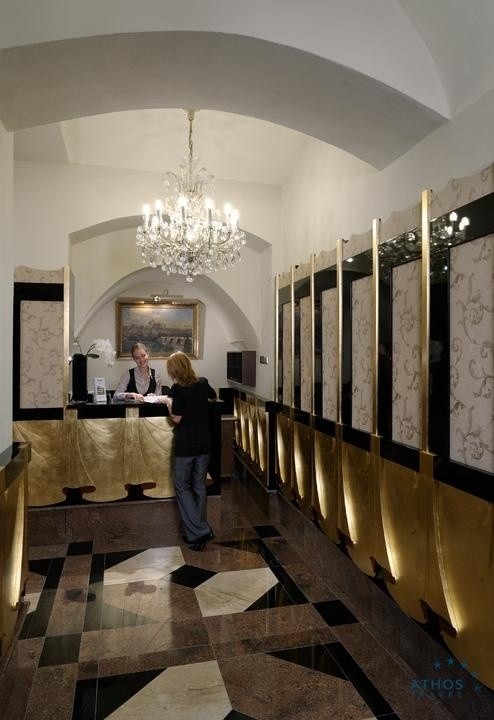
[112,342,163,402]
[155,350,217,551]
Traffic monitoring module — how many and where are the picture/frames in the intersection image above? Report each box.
[113,296,202,362]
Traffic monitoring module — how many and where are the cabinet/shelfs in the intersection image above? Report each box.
[220,414,240,478]
[226,350,256,388]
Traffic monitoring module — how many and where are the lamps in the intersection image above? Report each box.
[134,107,248,285]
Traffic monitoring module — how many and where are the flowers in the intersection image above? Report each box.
[73,335,117,368]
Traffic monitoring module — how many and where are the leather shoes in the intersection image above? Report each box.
[189,531,216,551]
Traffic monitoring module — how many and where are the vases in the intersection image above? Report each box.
[71,353,90,405]
